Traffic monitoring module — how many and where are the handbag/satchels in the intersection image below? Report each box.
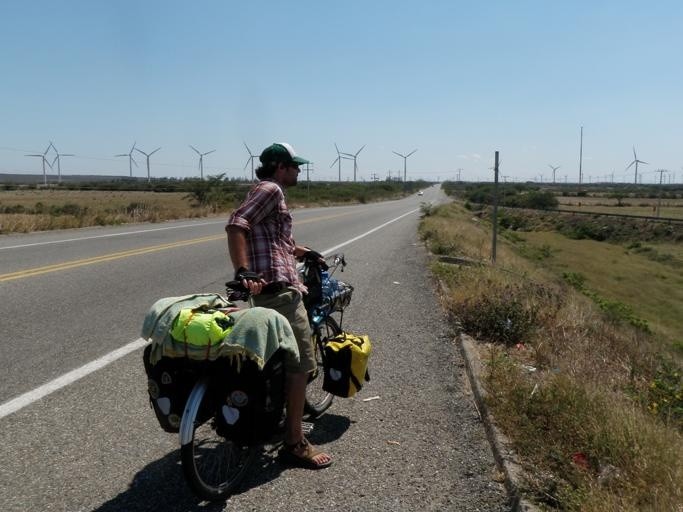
[211,349,284,446]
[322,331,371,397]
[142,343,214,432]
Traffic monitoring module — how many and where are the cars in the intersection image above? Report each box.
[431,184,435,187]
[416,190,423,196]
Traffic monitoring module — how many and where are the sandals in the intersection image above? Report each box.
[283,437,332,468]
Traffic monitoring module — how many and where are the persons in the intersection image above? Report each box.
[223,142,334,470]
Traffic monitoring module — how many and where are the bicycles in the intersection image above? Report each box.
[174,250,352,503]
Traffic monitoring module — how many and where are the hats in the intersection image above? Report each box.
[260,142,308,165]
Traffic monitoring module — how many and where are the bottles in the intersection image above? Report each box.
[318,271,333,297]
